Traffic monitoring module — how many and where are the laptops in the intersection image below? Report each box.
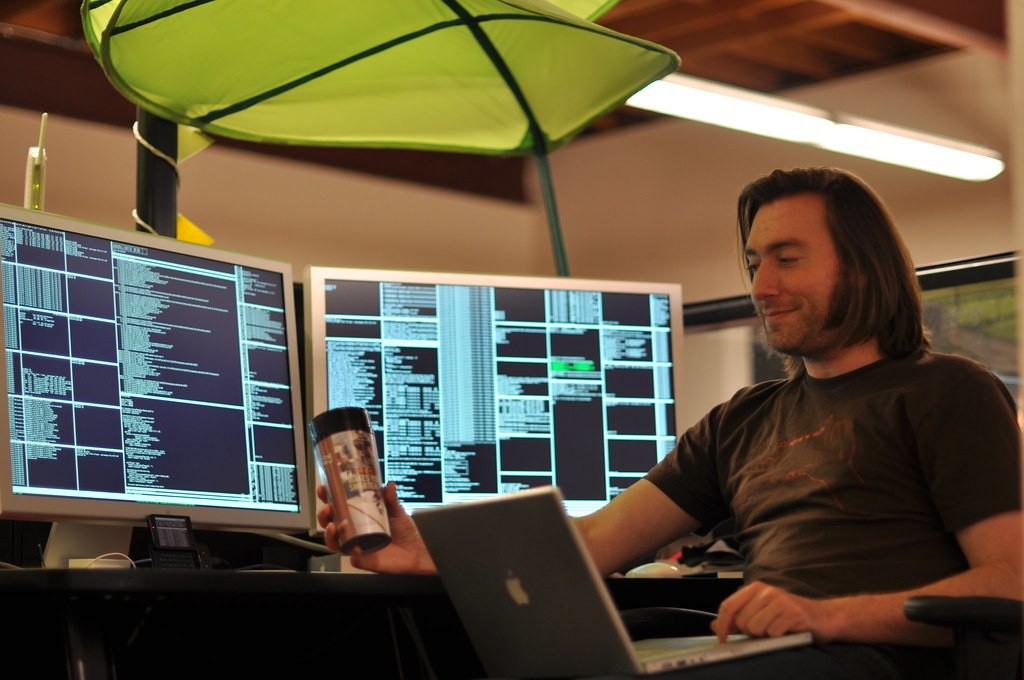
[410,484,815,680]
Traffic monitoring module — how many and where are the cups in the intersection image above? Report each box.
[309,407,391,555]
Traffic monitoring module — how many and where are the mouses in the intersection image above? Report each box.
[624,562,680,578]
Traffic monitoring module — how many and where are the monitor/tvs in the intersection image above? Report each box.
[302,263,683,519]
[1,202,312,567]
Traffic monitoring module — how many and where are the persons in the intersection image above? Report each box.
[318,165,1024,680]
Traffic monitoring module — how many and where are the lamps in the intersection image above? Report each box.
[626,69,1006,182]
